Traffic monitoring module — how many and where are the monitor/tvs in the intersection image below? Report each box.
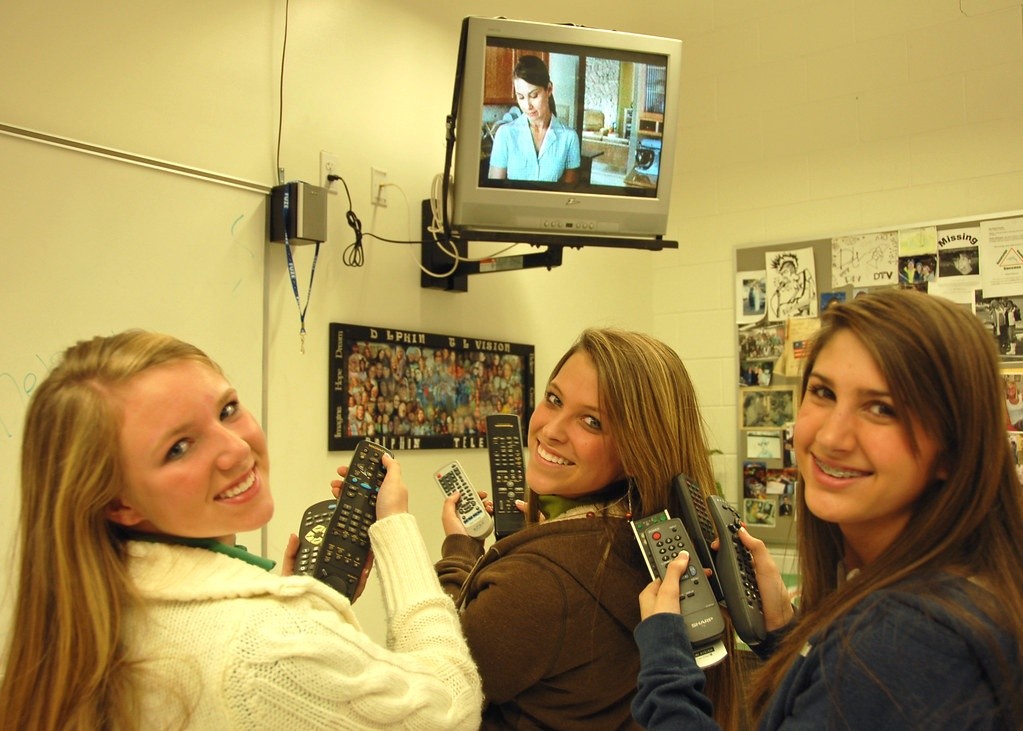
[436,17,682,239]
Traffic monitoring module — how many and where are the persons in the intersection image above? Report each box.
[487,54,581,183]
[348,342,523,435]
[740,281,866,524]
[630,290,1023,731]
[900,251,1023,464]
[436,327,736,731]
[0,328,484,731]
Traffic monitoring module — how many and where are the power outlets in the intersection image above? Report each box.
[318,149,338,194]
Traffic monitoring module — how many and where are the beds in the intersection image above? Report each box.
[581,109,629,168]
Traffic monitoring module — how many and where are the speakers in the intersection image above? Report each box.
[270,182,328,246]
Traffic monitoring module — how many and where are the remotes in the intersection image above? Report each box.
[630,473,768,669]
[487,412,528,540]
[313,440,395,606]
[295,499,341,576]
[433,459,494,540]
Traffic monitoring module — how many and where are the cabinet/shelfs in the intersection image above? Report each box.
[484,47,549,105]
